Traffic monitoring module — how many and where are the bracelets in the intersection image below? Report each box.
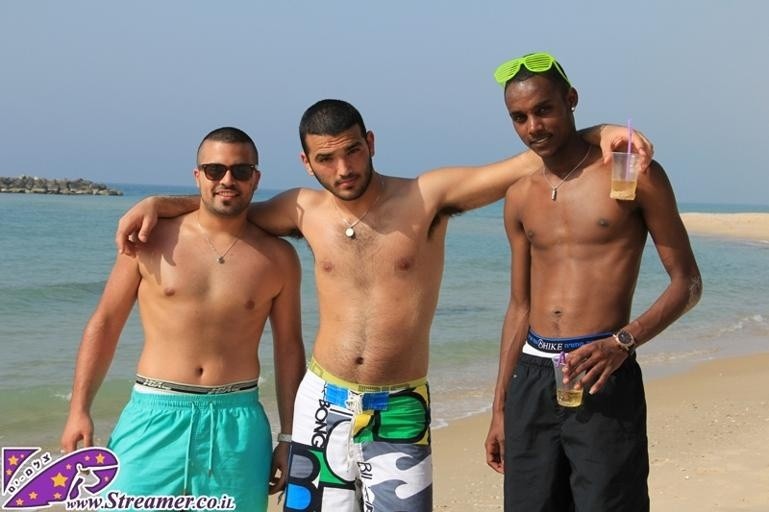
[277,434,293,442]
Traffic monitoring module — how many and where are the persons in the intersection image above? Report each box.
[62,127,307,511]
[116,99,654,512]
[484,52,703,512]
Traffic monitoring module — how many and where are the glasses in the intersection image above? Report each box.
[196,163,259,182]
[494,53,571,87]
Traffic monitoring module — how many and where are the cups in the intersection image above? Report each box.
[609,152,641,201]
[552,354,587,407]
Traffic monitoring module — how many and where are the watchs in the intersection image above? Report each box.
[613,328,636,355]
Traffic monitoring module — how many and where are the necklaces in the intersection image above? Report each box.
[195,212,244,264]
[330,173,385,239]
[544,145,592,201]
